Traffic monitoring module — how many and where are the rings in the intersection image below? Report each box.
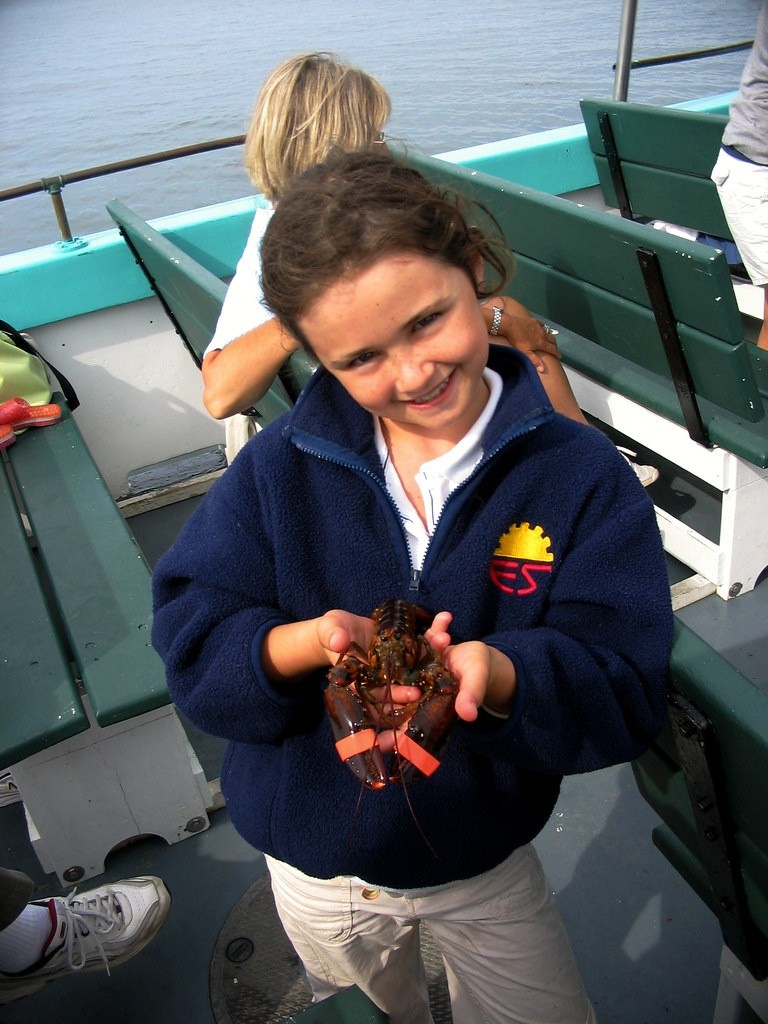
[544,324,552,334]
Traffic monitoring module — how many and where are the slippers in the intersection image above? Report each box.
[0,424,17,449]
[0,397,63,430]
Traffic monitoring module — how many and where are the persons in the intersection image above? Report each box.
[0,770,172,1005]
[151,52,675,1024]
[711,0,768,352]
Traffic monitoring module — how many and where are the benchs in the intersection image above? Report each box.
[0,98,768,1024]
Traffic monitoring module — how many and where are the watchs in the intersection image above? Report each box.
[490,306,503,335]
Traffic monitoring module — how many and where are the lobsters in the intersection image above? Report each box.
[324,597,459,789]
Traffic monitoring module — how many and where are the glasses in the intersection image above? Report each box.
[373,132,386,144]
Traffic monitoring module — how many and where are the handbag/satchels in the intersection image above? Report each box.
[0,319,79,434]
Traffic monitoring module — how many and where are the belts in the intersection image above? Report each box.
[721,144,768,167]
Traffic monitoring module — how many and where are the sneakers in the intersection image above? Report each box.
[0,876,172,1001]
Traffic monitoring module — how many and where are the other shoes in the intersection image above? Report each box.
[616,445,659,486]
